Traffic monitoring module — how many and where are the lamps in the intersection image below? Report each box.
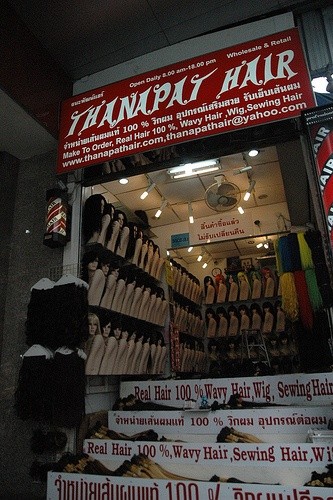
[154,198,169,218]
[256,239,271,248]
[140,174,156,200]
[233,159,252,176]
[197,247,207,262]
[244,179,257,202]
[236,202,244,215]
[202,254,213,269]
[188,200,194,224]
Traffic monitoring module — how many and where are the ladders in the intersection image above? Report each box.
[240,328,271,367]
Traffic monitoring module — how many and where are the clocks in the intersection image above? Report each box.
[211,268,222,277]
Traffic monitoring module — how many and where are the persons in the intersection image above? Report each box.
[262,268,274,296]
[204,276,215,304]
[82,194,164,279]
[250,271,262,299]
[262,302,274,333]
[251,303,261,334]
[83,250,167,327]
[237,272,251,301]
[215,274,227,303]
[238,304,249,335]
[274,300,285,331]
[167,262,205,373]
[227,271,238,301]
[205,307,216,337]
[228,305,238,336]
[206,332,290,361]
[216,307,227,337]
[86,310,167,375]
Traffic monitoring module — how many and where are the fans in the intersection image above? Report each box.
[204,175,240,212]
